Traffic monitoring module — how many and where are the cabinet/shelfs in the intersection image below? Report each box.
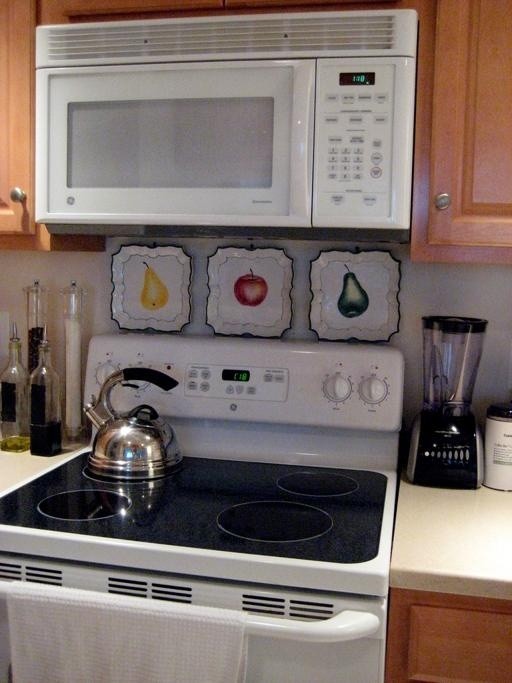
[400,0,511,270]
[0,0,112,257]
[379,466,510,681]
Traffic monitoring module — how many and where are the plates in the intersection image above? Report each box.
[203,245,294,338]
[307,246,402,340]
[107,240,193,332]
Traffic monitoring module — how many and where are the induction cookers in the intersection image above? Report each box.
[1,450,397,601]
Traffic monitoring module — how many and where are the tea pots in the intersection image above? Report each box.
[80,366,184,480]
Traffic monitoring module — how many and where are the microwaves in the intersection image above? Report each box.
[31,4,418,236]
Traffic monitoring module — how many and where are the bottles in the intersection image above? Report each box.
[59,278,83,443]
[0,323,31,452]
[30,321,63,457]
[24,277,49,377]
[482,403,512,493]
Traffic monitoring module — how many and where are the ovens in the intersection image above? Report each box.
[0,554,387,682]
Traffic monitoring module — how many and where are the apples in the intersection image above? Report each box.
[234,268,267,306]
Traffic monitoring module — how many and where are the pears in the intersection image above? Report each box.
[338,264,369,318]
[141,262,169,309]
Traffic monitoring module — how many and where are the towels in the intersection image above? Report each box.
[4,583,253,683]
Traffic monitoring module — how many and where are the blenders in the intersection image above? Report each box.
[398,314,488,489]
